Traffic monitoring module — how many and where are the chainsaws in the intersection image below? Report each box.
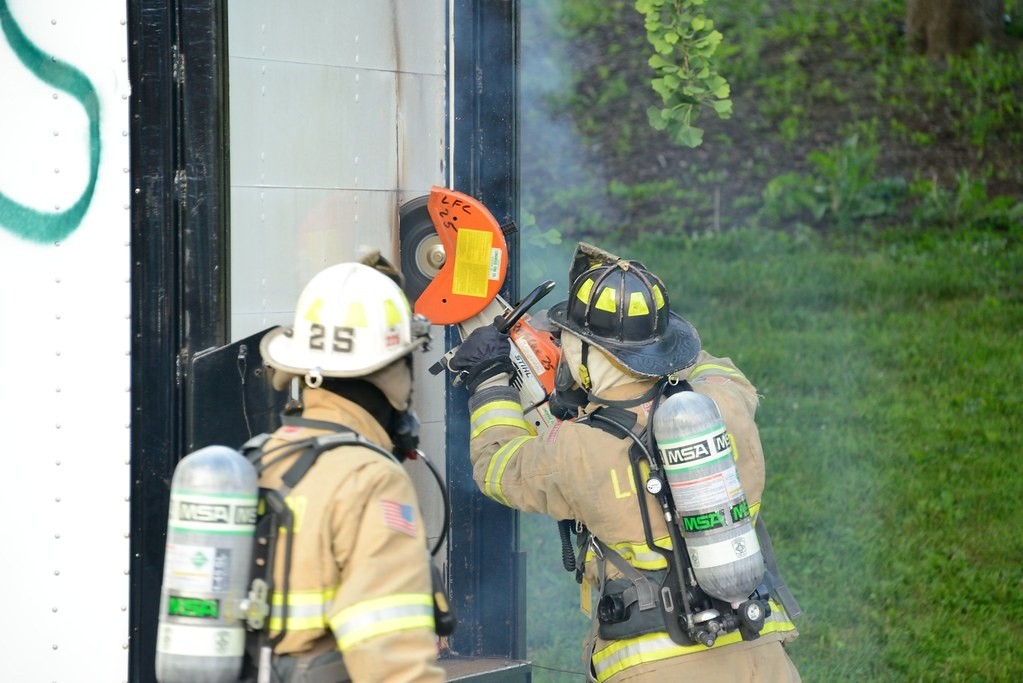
[401,184,592,571]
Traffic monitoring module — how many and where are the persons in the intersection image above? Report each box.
[236,263,447,683]
[450,260,802,683]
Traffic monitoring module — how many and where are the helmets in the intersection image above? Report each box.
[259,262,430,378]
[546,259,702,379]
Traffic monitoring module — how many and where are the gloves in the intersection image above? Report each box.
[447,324,517,396]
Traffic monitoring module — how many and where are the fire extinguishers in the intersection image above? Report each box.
[154,446,258,682]
[654,391,765,613]
